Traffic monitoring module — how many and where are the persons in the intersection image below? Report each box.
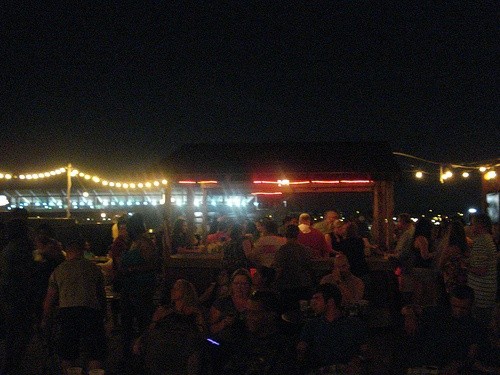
[0,208,500,375]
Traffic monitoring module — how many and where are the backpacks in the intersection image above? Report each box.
[124,252,166,298]
